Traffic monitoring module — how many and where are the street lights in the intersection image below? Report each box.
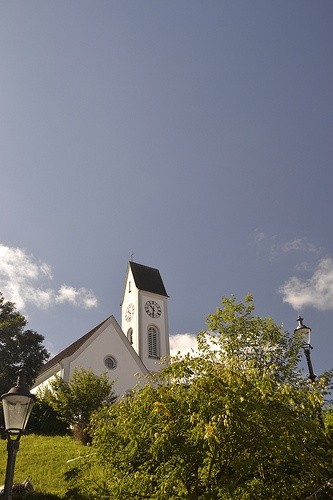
[293,313,327,434]
[1,368,38,499]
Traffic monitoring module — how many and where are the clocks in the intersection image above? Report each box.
[145,300,161,319]
[125,303,134,323]
